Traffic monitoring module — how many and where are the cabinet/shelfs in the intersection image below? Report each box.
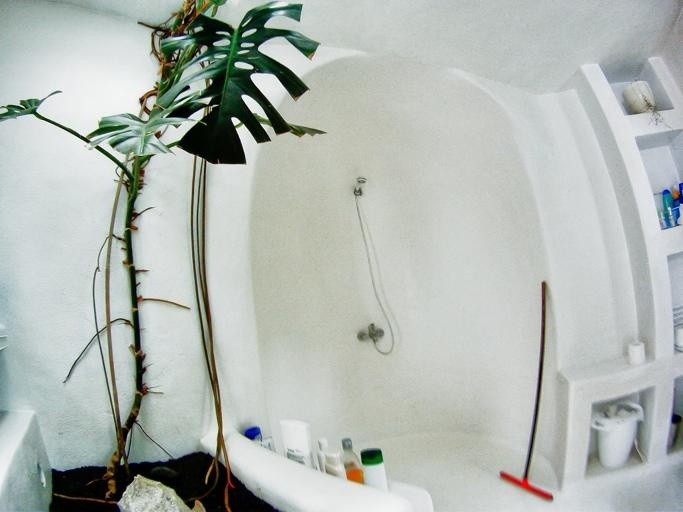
[552,56,682,491]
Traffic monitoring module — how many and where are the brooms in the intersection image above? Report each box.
[500,283,556,501]
